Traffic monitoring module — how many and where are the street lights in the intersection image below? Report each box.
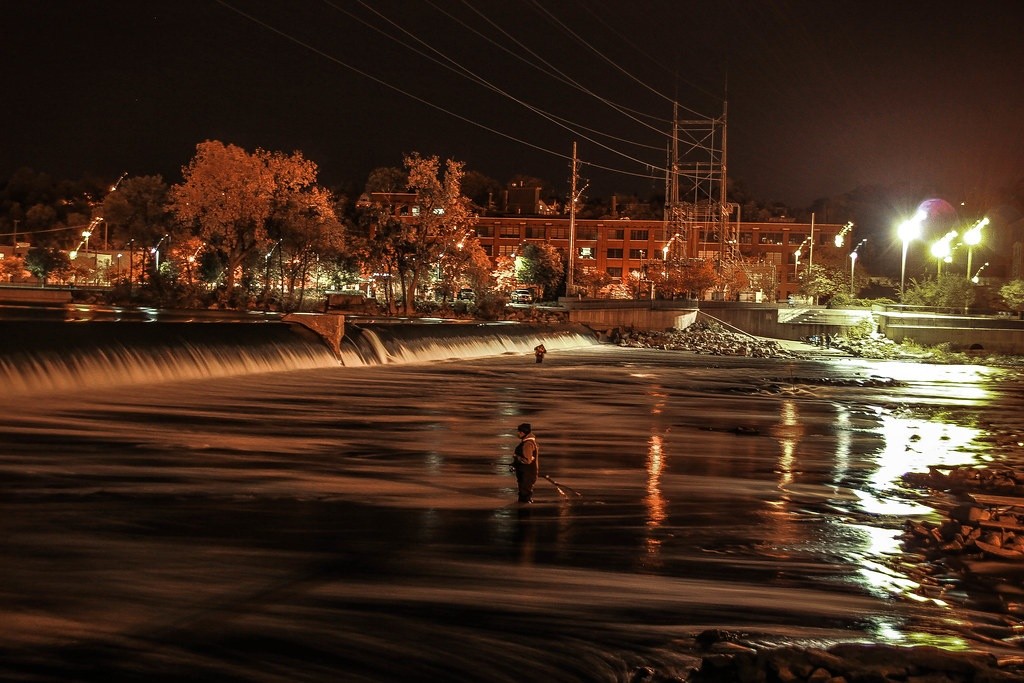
[898,224,915,310]
[963,230,979,316]
[82,232,90,285]
[849,251,857,292]
[118,252,122,286]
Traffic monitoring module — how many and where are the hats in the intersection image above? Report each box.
[518,423,531,434]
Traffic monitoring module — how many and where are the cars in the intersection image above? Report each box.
[457,288,476,301]
[511,290,532,304]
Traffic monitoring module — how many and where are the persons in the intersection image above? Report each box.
[805,335,809,344]
[732,297,735,304]
[815,332,831,349]
[534,344,547,363]
[509,423,539,503]
